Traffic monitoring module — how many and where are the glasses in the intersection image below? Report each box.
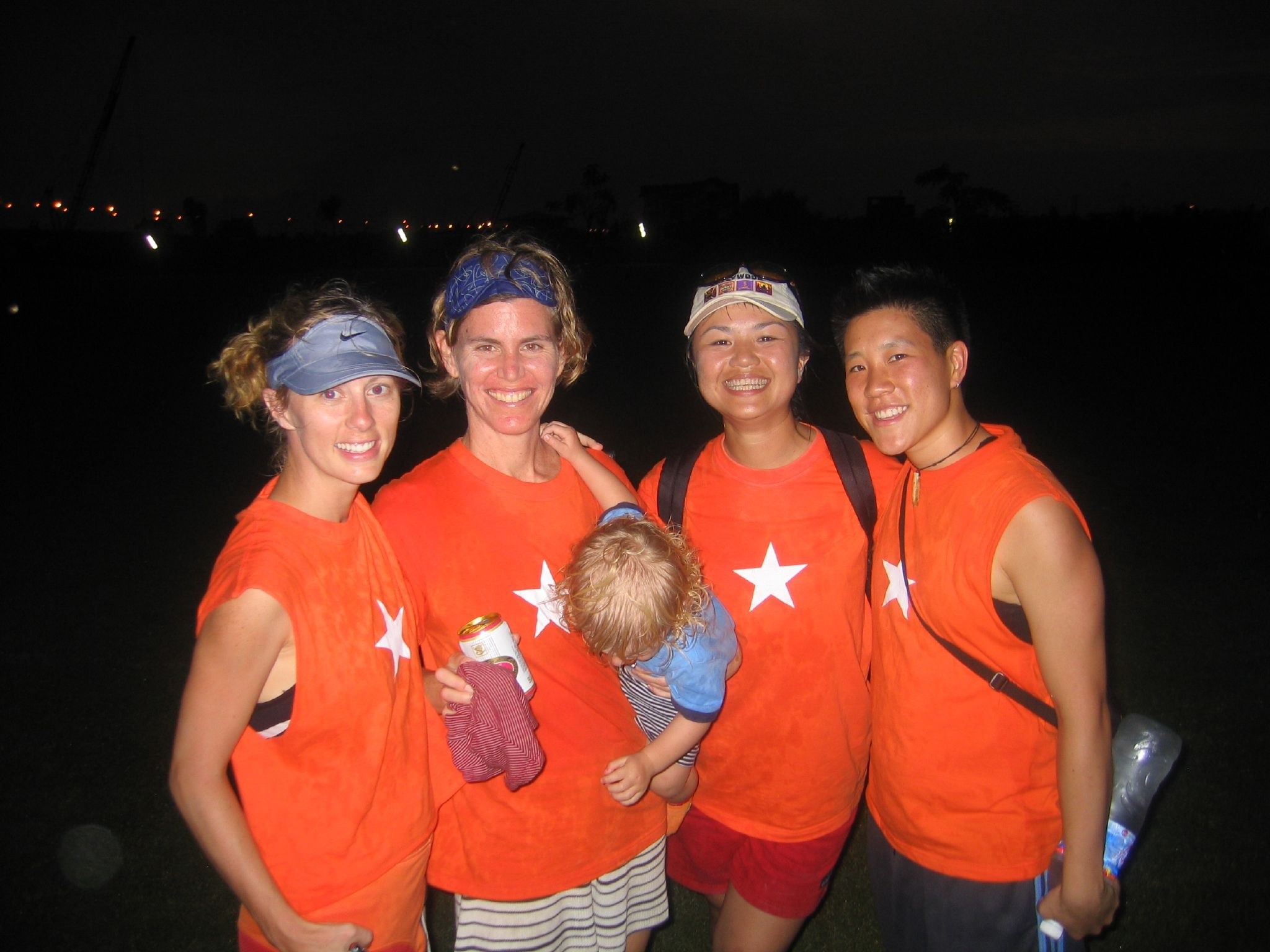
[686,269,807,349]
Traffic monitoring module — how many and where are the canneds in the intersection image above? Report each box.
[457,613,537,702]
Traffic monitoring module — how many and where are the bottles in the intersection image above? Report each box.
[1039,714,1182,939]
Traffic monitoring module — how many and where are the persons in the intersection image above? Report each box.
[637,259,900,952]
[369,241,741,952]
[541,420,737,806]
[835,267,1119,952]
[167,283,605,952]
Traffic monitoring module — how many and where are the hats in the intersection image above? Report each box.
[684,267,804,339]
[267,315,422,396]
[446,254,557,318]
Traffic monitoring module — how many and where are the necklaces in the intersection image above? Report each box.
[912,421,980,505]
